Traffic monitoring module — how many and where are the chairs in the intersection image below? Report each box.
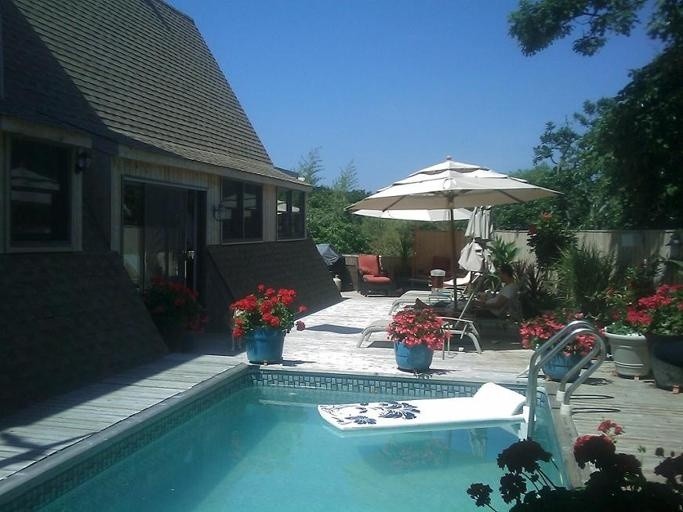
[356,271,521,353]
[318,382,537,441]
[356,253,392,297]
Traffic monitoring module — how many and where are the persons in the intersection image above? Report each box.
[410,264,518,319]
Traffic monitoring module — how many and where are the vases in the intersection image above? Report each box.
[604,326,649,377]
[533,341,583,382]
[241,326,285,365]
[641,328,682,392]
[159,336,188,354]
[394,338,433,372]
[333,275,342,291]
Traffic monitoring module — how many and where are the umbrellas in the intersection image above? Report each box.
[464,202,496,244]
[343,155,566,308]
[455,237,500,276]
[350,206,474,275]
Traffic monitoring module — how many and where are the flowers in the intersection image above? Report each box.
[141,275,201,336]
[519,299,610,356]
[390,303,453,350]
[596,278,683,339]
[228,277,310,338]
